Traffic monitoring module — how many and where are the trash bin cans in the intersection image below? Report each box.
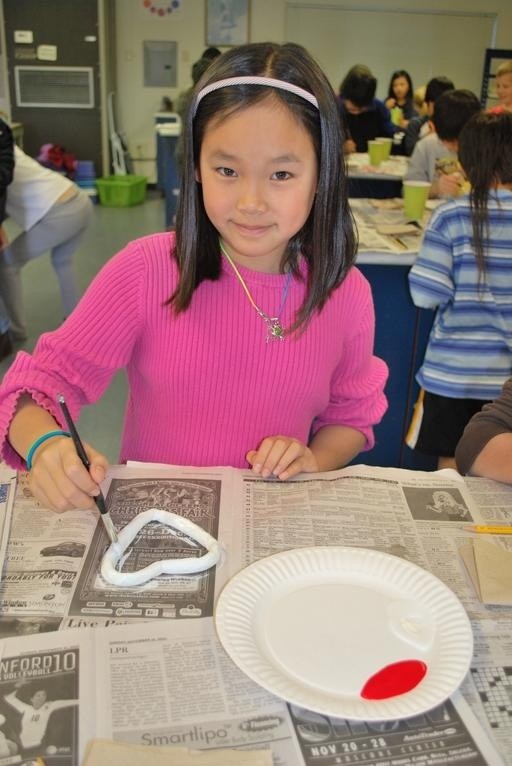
[153,112,184,199]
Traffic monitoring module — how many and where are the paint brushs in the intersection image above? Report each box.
[55,393,124,560]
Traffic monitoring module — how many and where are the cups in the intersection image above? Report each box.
[401,178,430,220]
[375,136,392,160]
[391,108,402,125]
[366,139,384,168]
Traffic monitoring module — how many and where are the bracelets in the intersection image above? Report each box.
[26,430,71,471]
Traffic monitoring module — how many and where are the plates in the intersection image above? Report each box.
[213,548,472,727]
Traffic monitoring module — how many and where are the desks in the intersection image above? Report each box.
[344,150,408,200]
[344,198,439,471]
[1,461,511,765]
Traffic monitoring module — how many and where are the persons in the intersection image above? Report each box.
[454,376,511,483]
[0,42,390,515]
[176,47,223,170]
[0,115,16,361]
[407,111,511,471]
[336,61,511,199]
[0,143,95,343]
[3,689,78,762]
[425,491,468,521]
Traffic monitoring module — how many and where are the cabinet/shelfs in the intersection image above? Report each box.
[480,48,511,109]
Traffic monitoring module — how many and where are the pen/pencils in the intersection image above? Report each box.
[459,524,512,535]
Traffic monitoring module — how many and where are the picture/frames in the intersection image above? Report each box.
[204,1,251,47]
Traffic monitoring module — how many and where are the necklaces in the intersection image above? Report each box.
[219,237,293,337]
